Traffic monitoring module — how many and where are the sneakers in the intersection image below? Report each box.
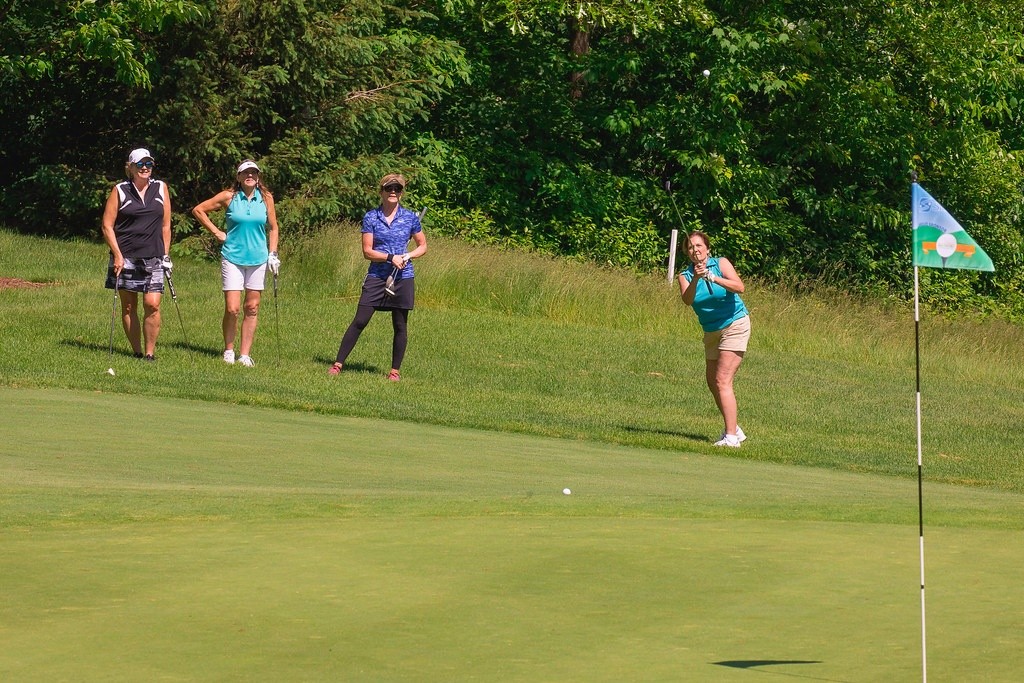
[238,355,255,366]
[716,427,748,442]
[387,371,401,381]
[713,435,741,446]
[327,364,342,376]
[224,350,235,363]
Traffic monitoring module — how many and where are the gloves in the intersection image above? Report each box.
[162,255,174,274]
[702,269,715,282]
[268,250,281,275]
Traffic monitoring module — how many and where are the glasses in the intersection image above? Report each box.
[384,186,403,192]
[134,162,153,167]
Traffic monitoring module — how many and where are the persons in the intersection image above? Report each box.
[330,173,429,381]
[101,147,173,362]
[677,230,751,447]
[192,159,281,366]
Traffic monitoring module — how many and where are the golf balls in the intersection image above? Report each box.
[703,70,710,77]
[562,488,571,495]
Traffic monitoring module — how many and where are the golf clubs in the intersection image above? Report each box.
[385,207,427,298]
[110,275,120,358]
[164,268,196,365]
[274,276,282,365]
[664,179,713,297]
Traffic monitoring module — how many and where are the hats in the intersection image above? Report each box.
[129,148,154,165]
[381,174,406,189]
[237,162,262,173]
